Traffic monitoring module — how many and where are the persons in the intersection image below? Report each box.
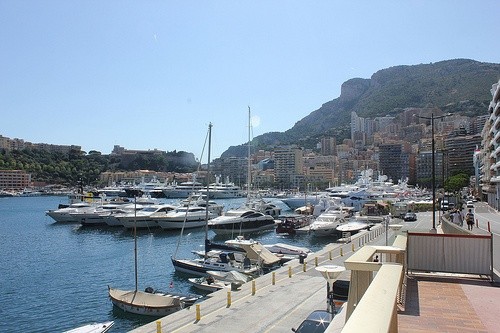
[466,209,475,231]
[244,255,250,269]
[450,208,464,227]
[300,251,304,264]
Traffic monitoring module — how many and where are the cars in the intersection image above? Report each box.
[467,201,473,207]
[292,310,335,333]
[404,213,416,221]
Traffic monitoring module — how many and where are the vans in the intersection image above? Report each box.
[441,201,449,210]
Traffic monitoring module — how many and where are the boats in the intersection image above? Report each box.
[192,238,280,269]
[63,321,114,333]
[207,205,281,234]
[171,256,251,278]
[144,286,200,308]
[107,285,184,316]
[96,176,240,197]
[46,189,173,228]
[151,194,216,228]
[189,278,225,291]
[275,204,374,239]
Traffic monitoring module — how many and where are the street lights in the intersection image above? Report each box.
[388,225,403,237]
[413,112,454,233]
[316,265,346,318]
